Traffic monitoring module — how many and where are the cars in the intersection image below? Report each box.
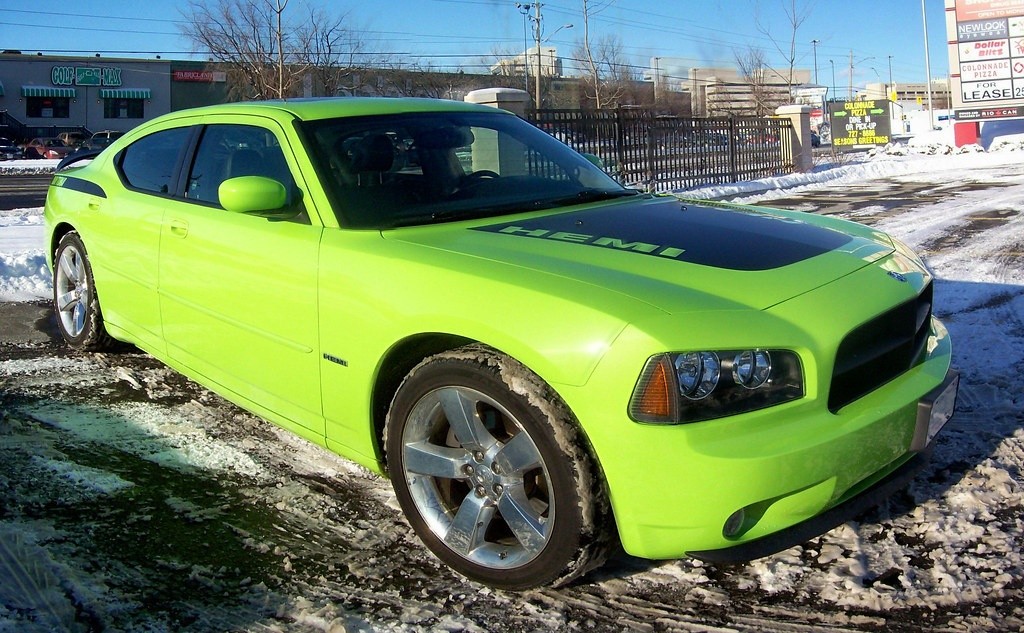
[810,130,821,147]
[386,125,777,168]
[0,128,125,162]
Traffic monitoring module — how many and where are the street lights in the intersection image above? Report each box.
[515,3,530,92]
[534,23,574,128]
[829,60,835,102]
[848,55,875,102]
[809,40,820,84]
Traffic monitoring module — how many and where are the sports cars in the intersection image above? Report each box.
[42,95,962,592]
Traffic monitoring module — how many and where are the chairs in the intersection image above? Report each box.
[226,125,469,223]
